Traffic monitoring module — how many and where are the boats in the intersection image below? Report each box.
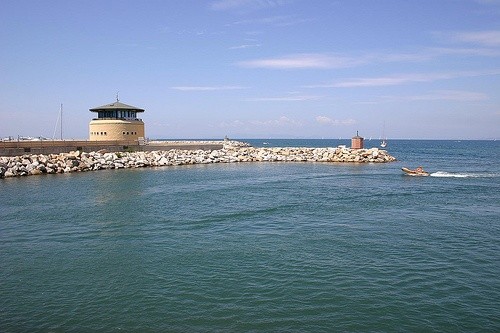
[401,166,430,176]
